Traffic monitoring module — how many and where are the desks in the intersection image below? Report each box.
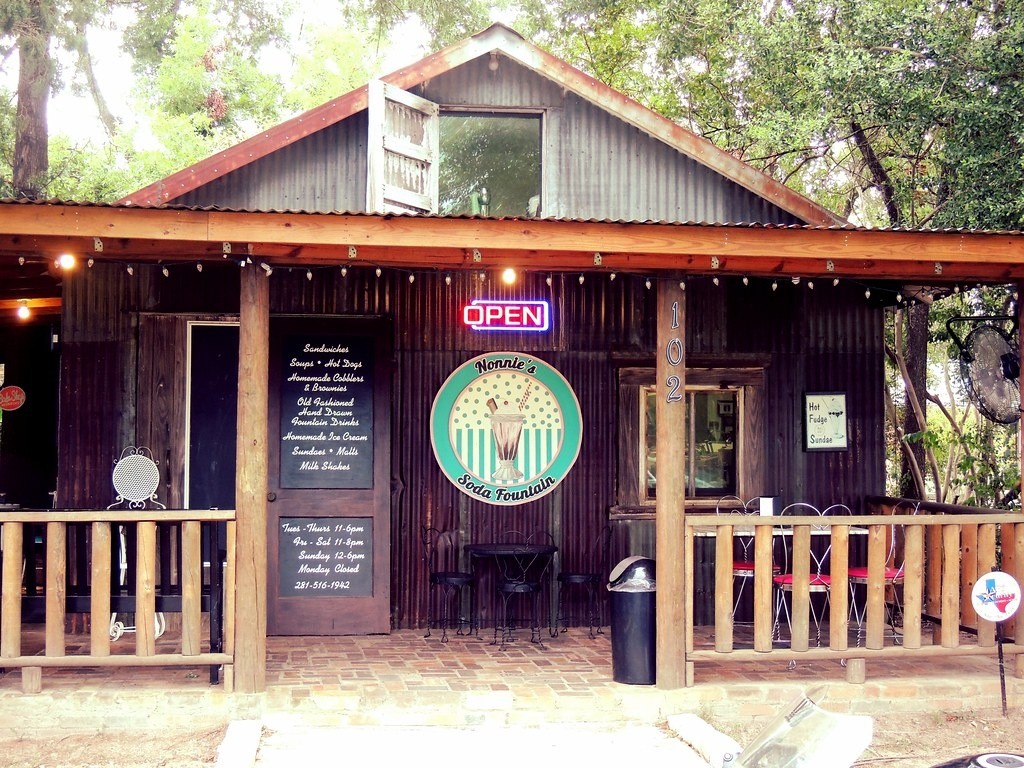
[694,525,868,643]
[464,543,558,643]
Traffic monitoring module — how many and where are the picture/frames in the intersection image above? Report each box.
[801,390,850,453]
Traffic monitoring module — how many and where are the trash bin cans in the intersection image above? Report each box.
[608,555,656,685]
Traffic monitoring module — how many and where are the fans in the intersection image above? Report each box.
[946,317,1020,424]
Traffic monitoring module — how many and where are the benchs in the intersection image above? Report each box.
[0,507,223,684]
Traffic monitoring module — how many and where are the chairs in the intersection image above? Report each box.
[711,495,792,644]
[421,525,478,643]
[848,500,922,647]
[772,503,853,643]
[552,525,614,639]
[491,531,555,652]
